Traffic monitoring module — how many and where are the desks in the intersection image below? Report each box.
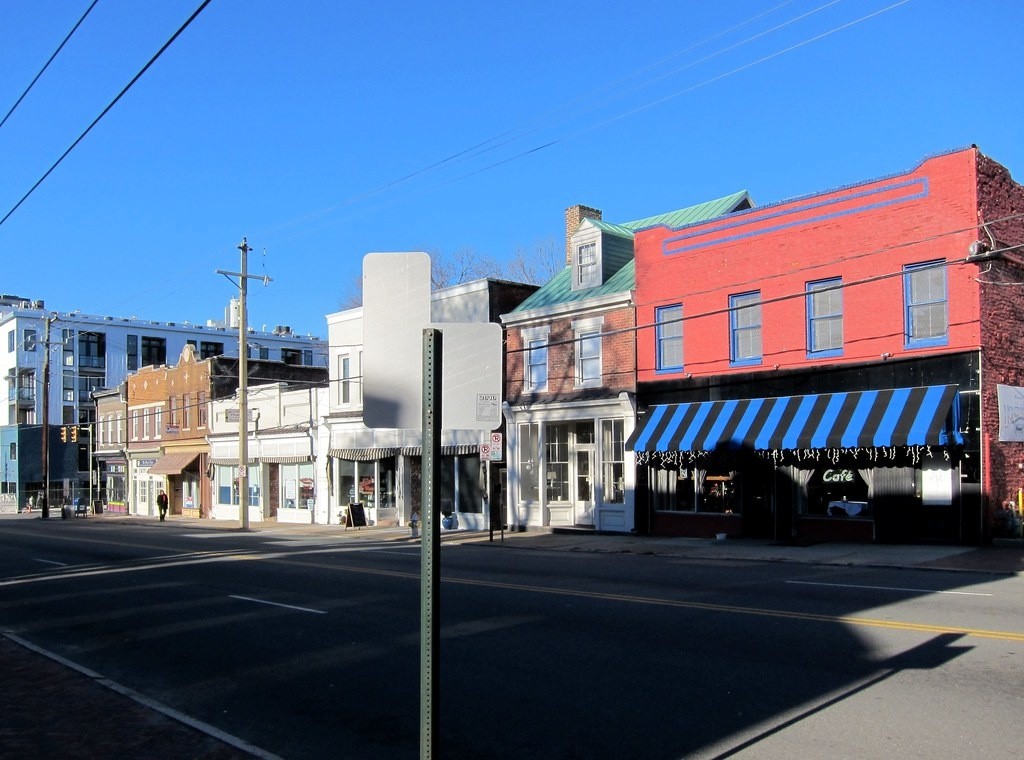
[828,501,868,517]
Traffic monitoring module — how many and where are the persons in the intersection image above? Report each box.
[157,490,168,522]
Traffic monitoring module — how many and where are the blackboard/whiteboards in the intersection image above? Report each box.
[346,502,367,527]
[92,500,103,514]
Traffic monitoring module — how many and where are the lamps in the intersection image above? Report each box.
[685,372,693,377]
[880,353,890,359]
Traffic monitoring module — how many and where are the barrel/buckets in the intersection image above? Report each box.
[716,533,727,540]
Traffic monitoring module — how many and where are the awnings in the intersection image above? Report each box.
[148,452,200,480]
[625,384,965,463]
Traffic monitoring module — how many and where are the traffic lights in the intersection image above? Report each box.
[60,426,67,442]
[70,426,76,443]
